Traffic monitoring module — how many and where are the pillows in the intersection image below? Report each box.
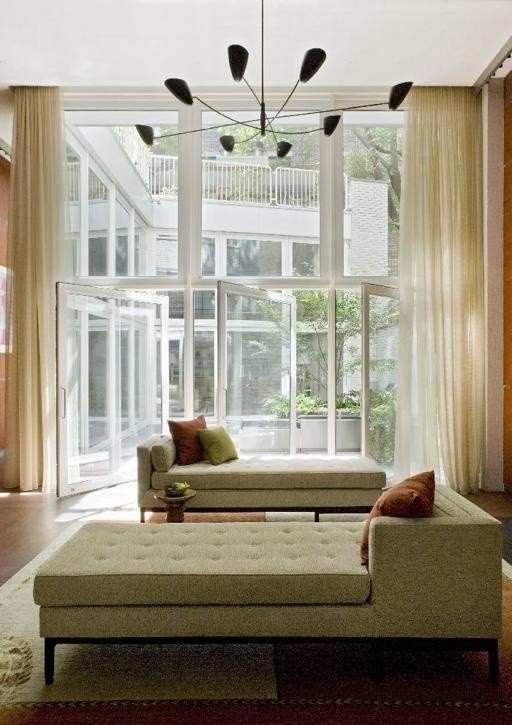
[151,435,177,472]
[357,470,436,568]
[167,414,213,466]
[194,425,240,465]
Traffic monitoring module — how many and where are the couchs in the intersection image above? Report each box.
[31,477,507,683]
[135,433,387,529]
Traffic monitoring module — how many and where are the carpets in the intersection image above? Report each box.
[1,510,511,712]
[491,514,511,567]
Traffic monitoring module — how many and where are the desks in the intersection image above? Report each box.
[153,486,199,523]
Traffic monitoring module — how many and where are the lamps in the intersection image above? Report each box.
[135,0,411,166]
